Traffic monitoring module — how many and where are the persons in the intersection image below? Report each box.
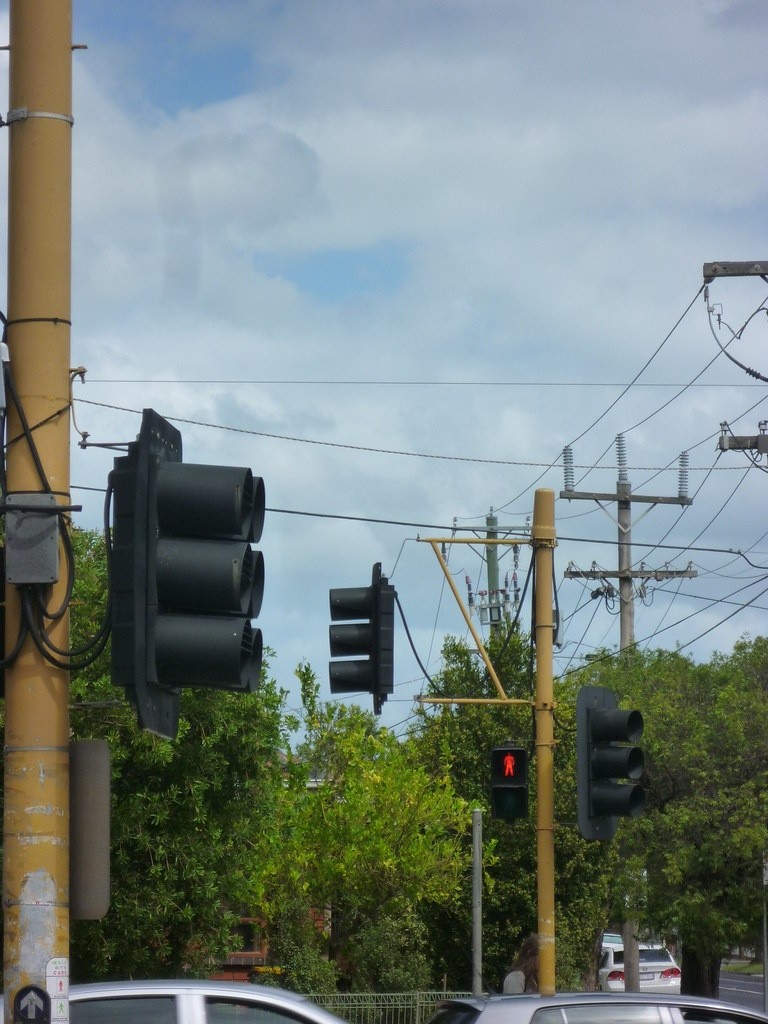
[503,935,540,995]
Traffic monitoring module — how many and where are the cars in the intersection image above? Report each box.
[0,979,349,1024]
[419,991,768,1024]
[595,944,683,995]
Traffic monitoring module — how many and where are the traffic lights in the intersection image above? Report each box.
[144,451,266,693]
[492,745,530,824]
[586,705,648,821]
[330,561,396,716]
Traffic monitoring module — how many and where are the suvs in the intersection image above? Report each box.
[601,932,624,948]
